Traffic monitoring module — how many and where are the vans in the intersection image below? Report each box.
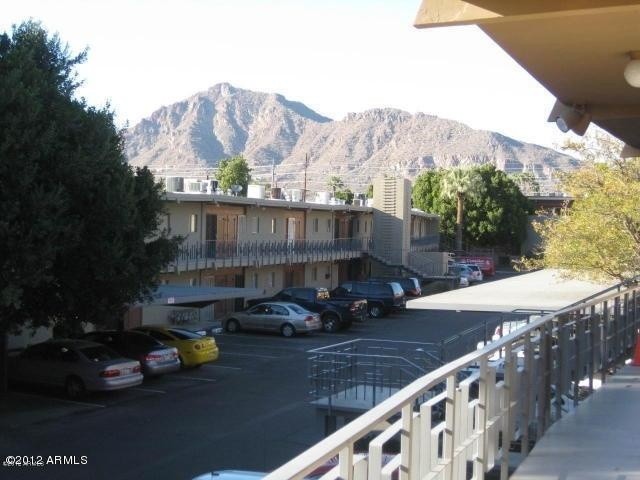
[448,256,496,286]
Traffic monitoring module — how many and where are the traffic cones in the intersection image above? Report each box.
[629,329,640,366]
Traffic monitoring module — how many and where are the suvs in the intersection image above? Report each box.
[222,275,421,337]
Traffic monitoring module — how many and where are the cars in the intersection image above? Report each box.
[2,325,220,398]
[190,447,398,479]
[462,310,603,448]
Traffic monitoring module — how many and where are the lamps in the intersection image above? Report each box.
[624,49,640,88]
